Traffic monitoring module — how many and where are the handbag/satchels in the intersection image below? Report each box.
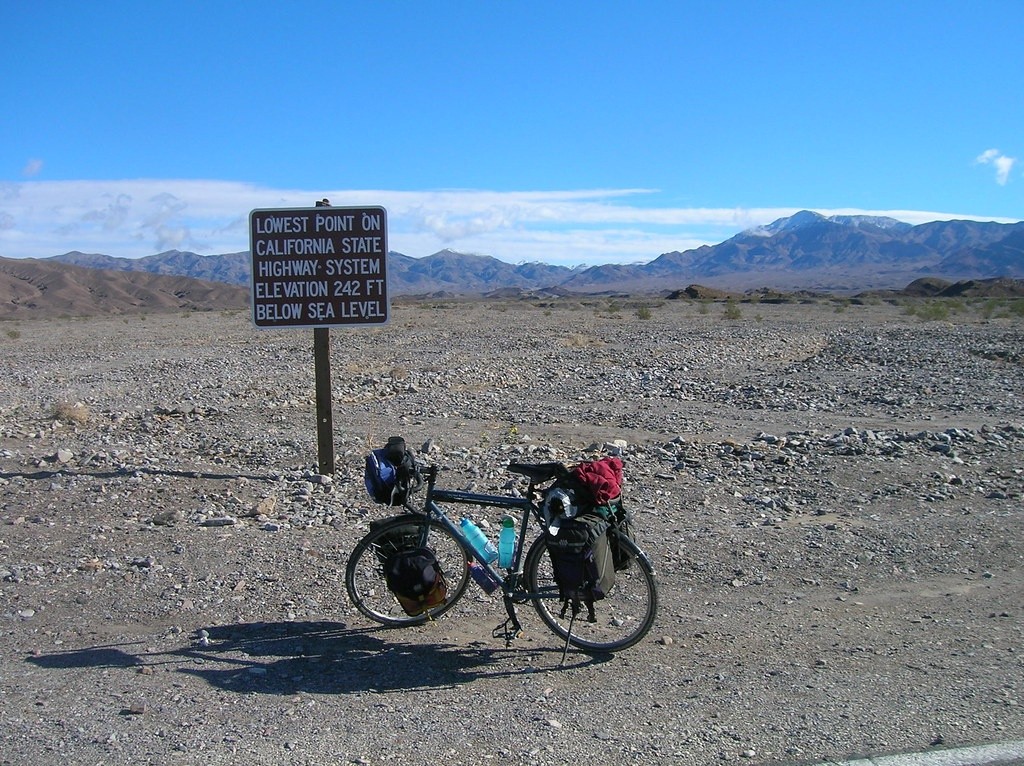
[544,514,615,622]
[384,544,447,617]
[609,509,636,572]
[364,436,416,508]
[370,515,424,563]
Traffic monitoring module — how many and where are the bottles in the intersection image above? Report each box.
[467,561,498,595]
[458,517,499,564]
[498,517,514,568]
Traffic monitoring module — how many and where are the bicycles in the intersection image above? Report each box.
[346,450,658,666]
